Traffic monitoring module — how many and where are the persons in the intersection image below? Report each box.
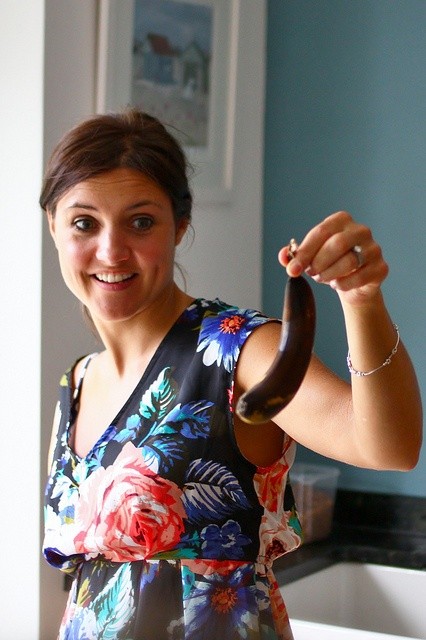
[43,110,423,640]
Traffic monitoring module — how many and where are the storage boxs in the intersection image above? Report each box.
[289,466,340,543]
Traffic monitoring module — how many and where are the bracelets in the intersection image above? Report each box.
[346,323,400,376]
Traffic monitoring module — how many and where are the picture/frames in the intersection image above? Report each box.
[97,2,242,209]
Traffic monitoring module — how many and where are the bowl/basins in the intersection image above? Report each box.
[278,464,340,539]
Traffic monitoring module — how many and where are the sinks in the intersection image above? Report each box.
[272,540,426,640]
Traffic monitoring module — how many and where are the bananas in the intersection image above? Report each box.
[235,236,317,425]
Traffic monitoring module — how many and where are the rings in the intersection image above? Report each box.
[352,244,364,269]
